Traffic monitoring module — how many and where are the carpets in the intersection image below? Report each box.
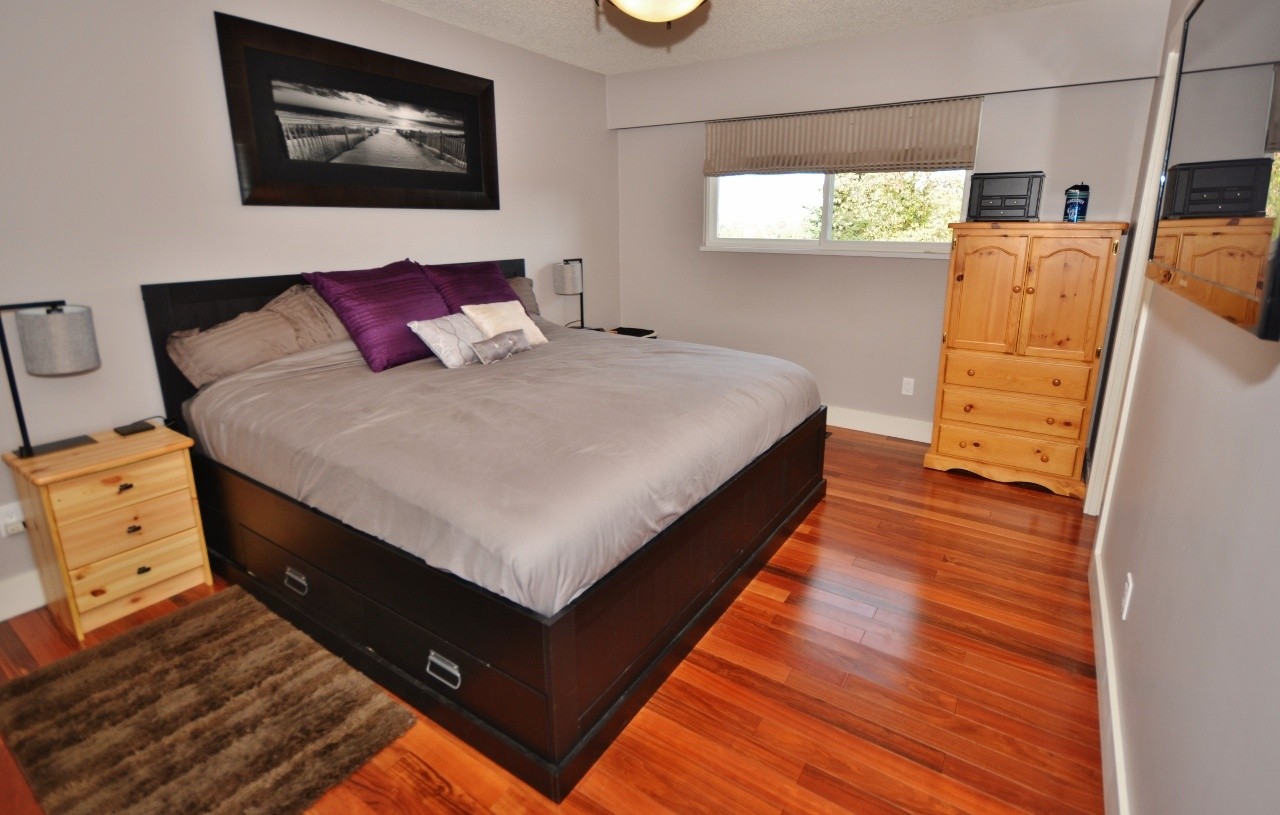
[0,583,420,815]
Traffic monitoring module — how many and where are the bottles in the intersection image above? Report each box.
[1063,184,1090,222]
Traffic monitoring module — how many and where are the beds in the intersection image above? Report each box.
[136,256,830,806]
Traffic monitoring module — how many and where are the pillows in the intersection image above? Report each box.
[166,258,552,391]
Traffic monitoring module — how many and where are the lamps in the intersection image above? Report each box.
[551,257,593,331]
[0,299,101,456]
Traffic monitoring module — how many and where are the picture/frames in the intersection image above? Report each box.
[213,10,500,210]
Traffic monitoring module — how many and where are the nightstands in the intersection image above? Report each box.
[1,420,216,640]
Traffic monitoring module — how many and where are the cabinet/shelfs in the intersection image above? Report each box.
[921,221,1130,500]
